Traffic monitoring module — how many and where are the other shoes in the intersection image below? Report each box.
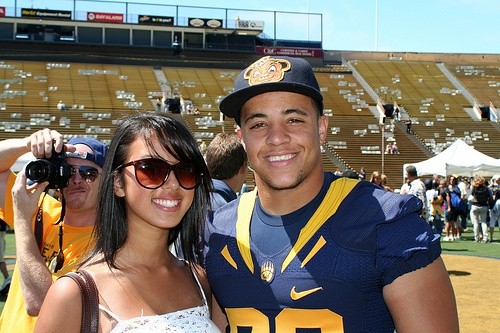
[441,227,494,244]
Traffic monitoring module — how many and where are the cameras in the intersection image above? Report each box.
[26,143,72,189]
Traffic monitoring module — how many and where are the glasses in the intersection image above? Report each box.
[116,159,204,190]
[67,166,103,184]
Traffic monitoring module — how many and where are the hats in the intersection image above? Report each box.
[219,56,323,118]
[62,136,108,169]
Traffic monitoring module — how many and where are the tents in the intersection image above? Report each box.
[401,136,497,192]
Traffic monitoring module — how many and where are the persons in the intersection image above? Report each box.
[404,119,412,135]
[164,128,253,271]
[31,111,223,333]
[0,127,118,333]
[328,166,500,247]
[185,103,201,116]
[1,202,14,293]
[203,55,461,333]
[383,141,400,156]
[163,96,171,113]
[466,174,494,245]
[155,95,162,114]
